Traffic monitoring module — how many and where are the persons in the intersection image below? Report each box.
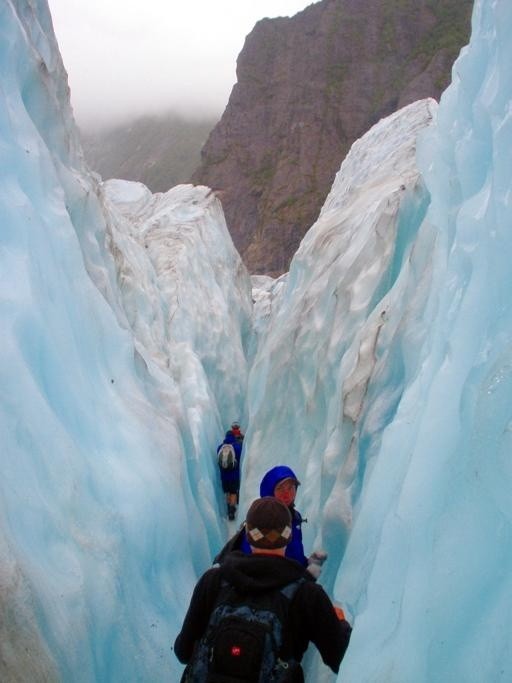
[231,421,244,505]
[173,496,353,683]
[240,464,327,579]
[216,430,242,520]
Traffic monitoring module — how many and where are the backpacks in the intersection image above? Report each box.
[217,442,237,471]
[185,566,305,682]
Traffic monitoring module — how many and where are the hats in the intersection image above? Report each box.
[246,496,292,548]
